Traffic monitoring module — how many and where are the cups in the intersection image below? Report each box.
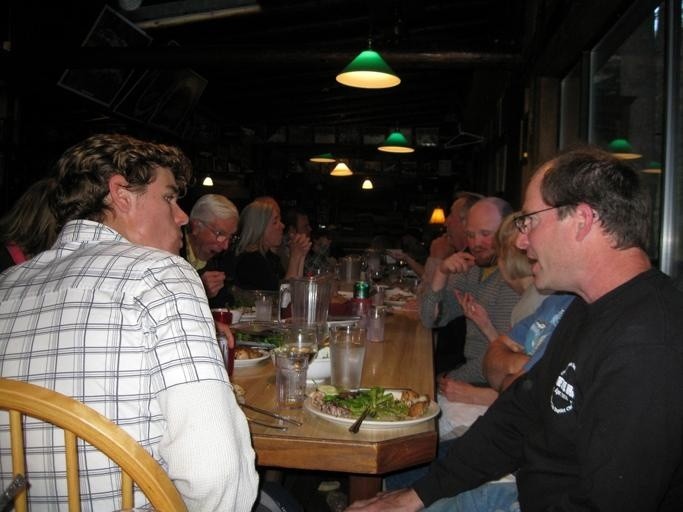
[330,325,368,388]
[289,278,331,344]
[350,300,367,320]
[369,285,384,308]
[273,353,309,409]
[368,309,385,343]
[255,297,273,323]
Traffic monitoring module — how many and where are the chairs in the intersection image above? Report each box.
[1,376,190,512]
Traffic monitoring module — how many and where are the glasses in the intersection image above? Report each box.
[198,217,242,244]
[511,201,580,234]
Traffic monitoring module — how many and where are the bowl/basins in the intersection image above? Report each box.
[279,358,331,385]
[211,309,241,324]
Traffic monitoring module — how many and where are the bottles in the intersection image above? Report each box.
[354,280,368,299]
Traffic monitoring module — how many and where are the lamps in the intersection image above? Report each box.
[431,207,446,225]
[331,163,355,176]
[336,49,400,91]
[608,139,643,160]
[378,132,416,153]
[639,160,664,176]
[312,152,338,163]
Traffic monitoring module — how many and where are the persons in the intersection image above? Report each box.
[287,201,336,279]
[0,131,304,512]
[418,196,523,387]
[234,195,312,300]
[382,295,593,493]
[384,244,437,280]
[431,211,554,437]
[337,145,683,511]
[178,187,243,308]
[415,190,484,284]
[0,174,69,270]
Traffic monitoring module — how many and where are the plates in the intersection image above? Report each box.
[383,300,407,309]
[304,387,441,430]
[233,349,271,368]
[240,314,255,321]
[326,317,355,330]
